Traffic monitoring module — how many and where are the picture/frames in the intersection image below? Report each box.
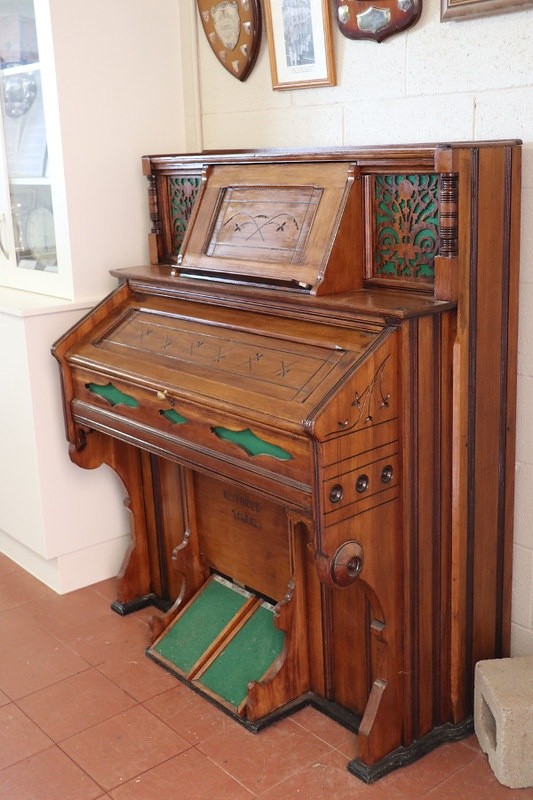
[264,0,337,91]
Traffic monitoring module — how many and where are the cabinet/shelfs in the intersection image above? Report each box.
[0,0,185,595]
